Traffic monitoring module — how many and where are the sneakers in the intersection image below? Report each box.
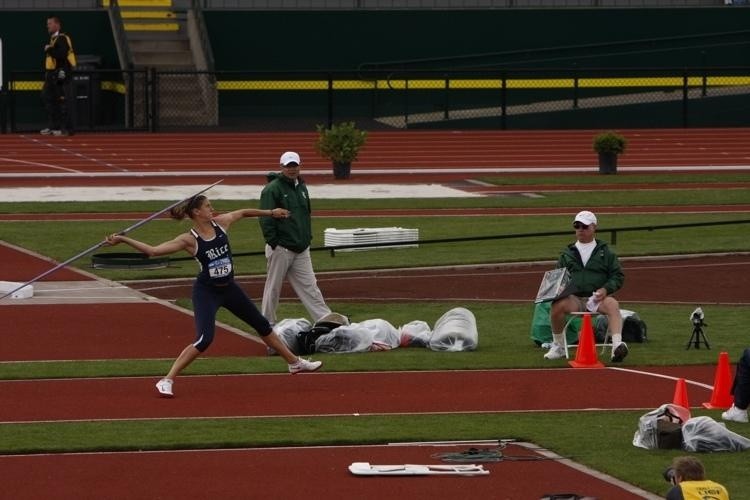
[543,345,566,360]
[39,127,53,136]
[722,402,749,424]
[52,129,68,136]
[610,341,629,362]
[156,378,174,398]
[287,356,322,374]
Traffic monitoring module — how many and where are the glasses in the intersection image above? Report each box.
[573,222,588,229]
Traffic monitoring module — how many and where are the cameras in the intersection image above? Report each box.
[663,467,677,485]
[689,307,707,326]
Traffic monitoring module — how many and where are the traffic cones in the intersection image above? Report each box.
[568,315,607,371]
[702,352,735,412]
[671,378,691,427]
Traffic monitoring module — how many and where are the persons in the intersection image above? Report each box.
[255,151,334,326]
[544,210,631,362]
[39,17,76,137]
[665,456,729,499]
[106,194,323,397]
[721,347,750,423]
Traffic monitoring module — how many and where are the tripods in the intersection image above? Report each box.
[686,327,711,351]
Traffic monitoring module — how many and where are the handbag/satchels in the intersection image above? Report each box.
[650,408,683,450]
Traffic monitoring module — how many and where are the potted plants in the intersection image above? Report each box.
[592,130,627,176]
[312,121,366,179]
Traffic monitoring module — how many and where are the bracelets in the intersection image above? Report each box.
[269,209,274,217]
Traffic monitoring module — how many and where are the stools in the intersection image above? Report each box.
[562,310,616,358]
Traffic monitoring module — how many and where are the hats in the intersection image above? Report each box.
[279,151,302,166]
[574,210,598,227]
